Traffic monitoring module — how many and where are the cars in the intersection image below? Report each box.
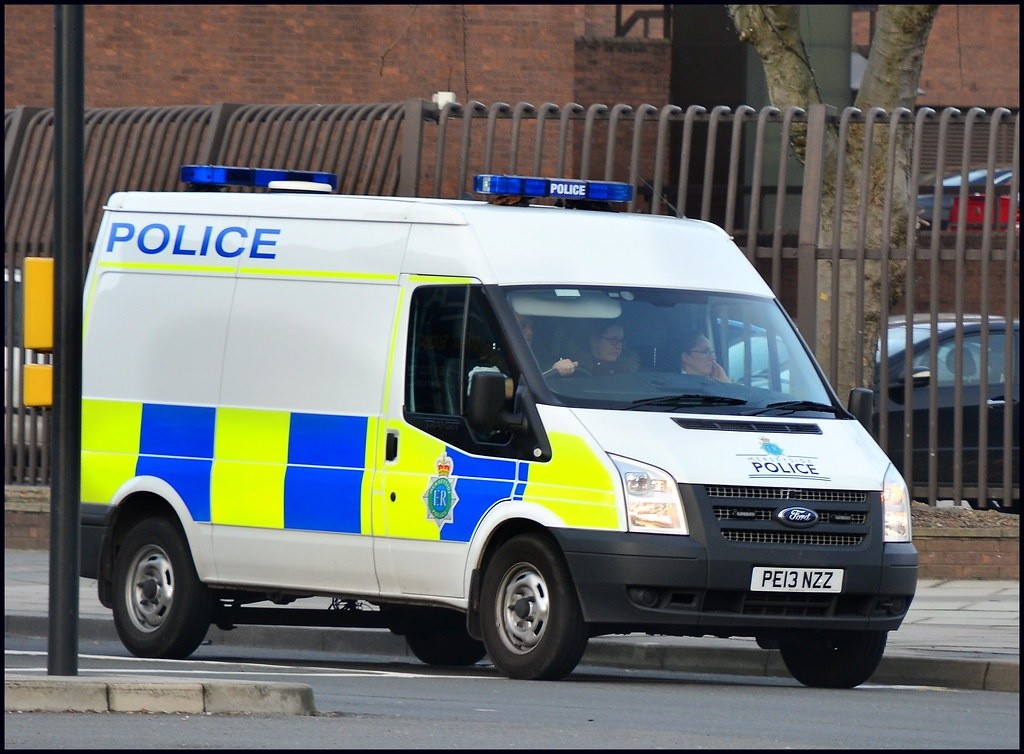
[916,160,1020,235]
[741,310,1016,393]
[850,319,1022,511]
[4,268,56,477]
[714,317,789,380]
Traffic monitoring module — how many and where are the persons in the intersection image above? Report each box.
[480,306,581,378]
[557,319,647,381]
[665,331,739,386]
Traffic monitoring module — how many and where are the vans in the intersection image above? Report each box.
[80,162,921,691]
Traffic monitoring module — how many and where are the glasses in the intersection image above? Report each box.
[690,348,716,358]
[599,335,625,345]
